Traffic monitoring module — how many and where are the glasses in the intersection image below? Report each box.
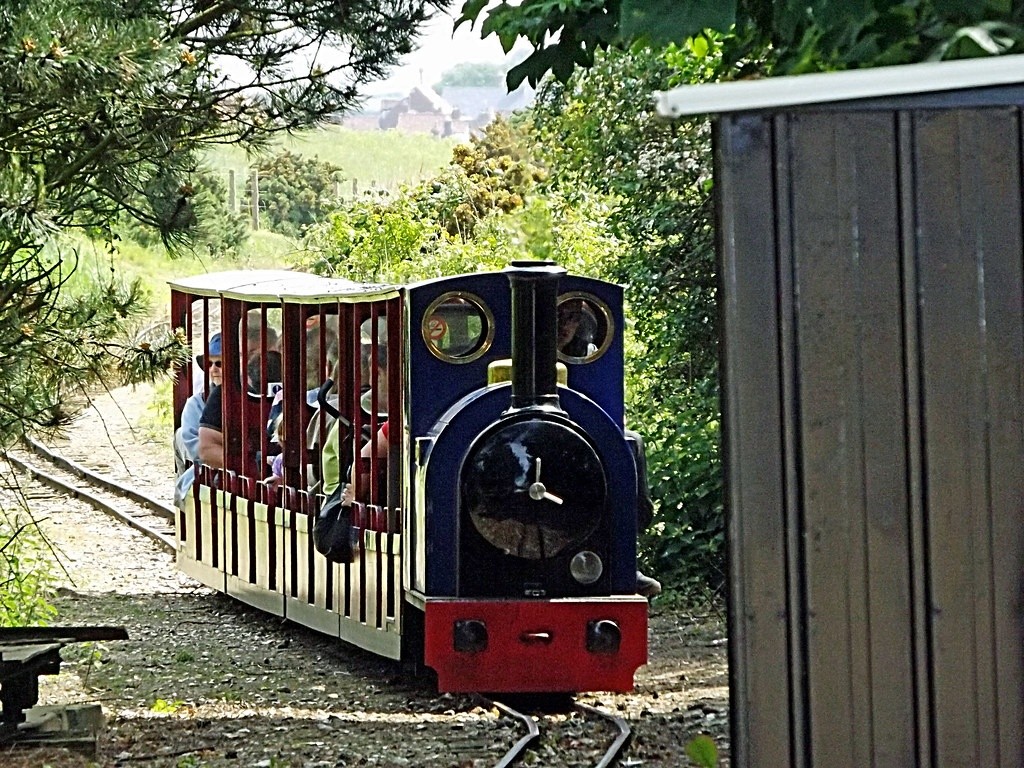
[209,361,221,367]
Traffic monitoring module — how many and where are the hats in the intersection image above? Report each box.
[196,333,221,371]
[360,316,387,346]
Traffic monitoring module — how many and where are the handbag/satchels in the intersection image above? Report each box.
[313,483,354,563]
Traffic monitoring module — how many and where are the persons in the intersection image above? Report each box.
[558,299,598,358]
[174,311,389,514]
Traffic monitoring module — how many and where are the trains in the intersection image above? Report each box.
[165,259,663,700]
